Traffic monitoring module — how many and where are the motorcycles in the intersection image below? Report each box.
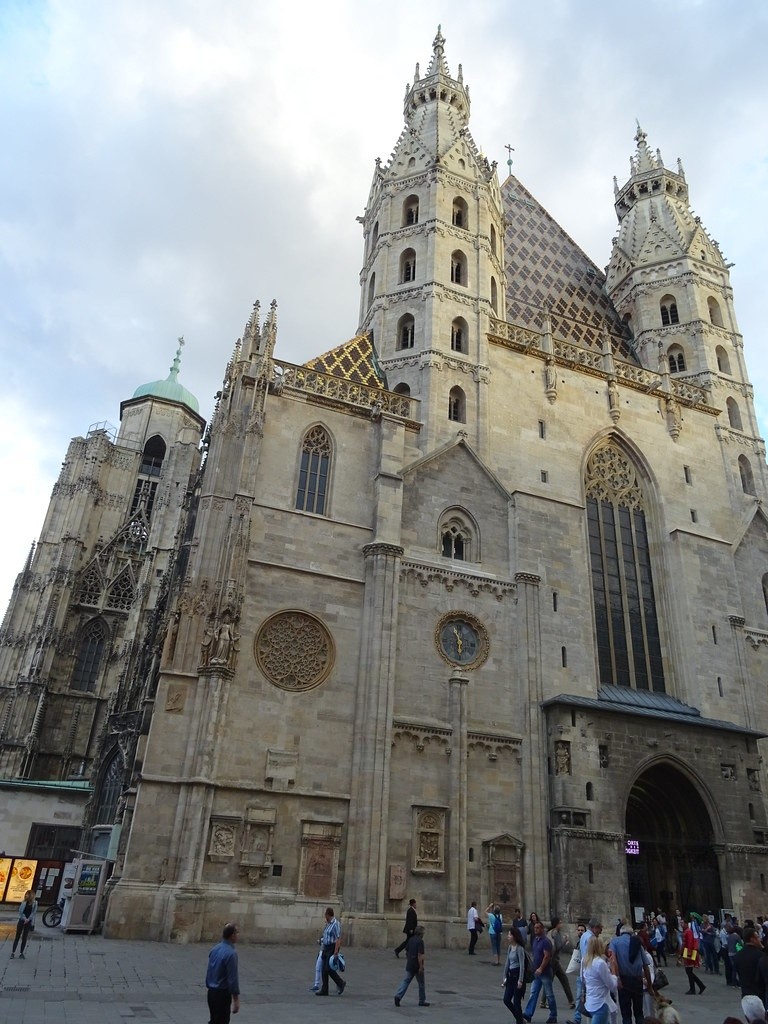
[42,897,66,927]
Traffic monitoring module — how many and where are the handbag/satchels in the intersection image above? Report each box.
[576,999,592,1018]
[30,925,35,931]
[565,949,582,976]
[652,968,669,991]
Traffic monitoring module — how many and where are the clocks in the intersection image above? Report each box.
[432,610,490,672]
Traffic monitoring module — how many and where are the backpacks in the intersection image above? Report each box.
[516,945,536,983]
[490,914,502,933]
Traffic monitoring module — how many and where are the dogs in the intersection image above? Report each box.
[652,991,682,1024]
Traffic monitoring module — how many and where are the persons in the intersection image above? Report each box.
[393,925,431,1007]
[608,381,621,410]
[205,923,240,1024]
[465,901,485,956]
[485,902,504,966]
[546,361,557,389]
[310,907,347,997]
[503,908,768,1024]
[393,899,418,959]
[667,399,677,415]
[10,889,38,960]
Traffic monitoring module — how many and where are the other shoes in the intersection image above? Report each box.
[522,1013,531,1023]
[699,985,706,995]
[310,986,319,990]
[19,954,26,959]
[419,1002,430,1006]
[540,1001,547,1008]
[685,990,695,995]
[491,962,500,966]
[395,996,400,1007]
[546,1017,557,1024]
[315,990,329,996]
[394,949,399,958]
[715,971,722,976]
[570,1002,575,1008]
[710,970,714,974]
[469,952,476,955]
[338,981,346,995]
[566,1020,575,1024]
[10,954,15,959]
